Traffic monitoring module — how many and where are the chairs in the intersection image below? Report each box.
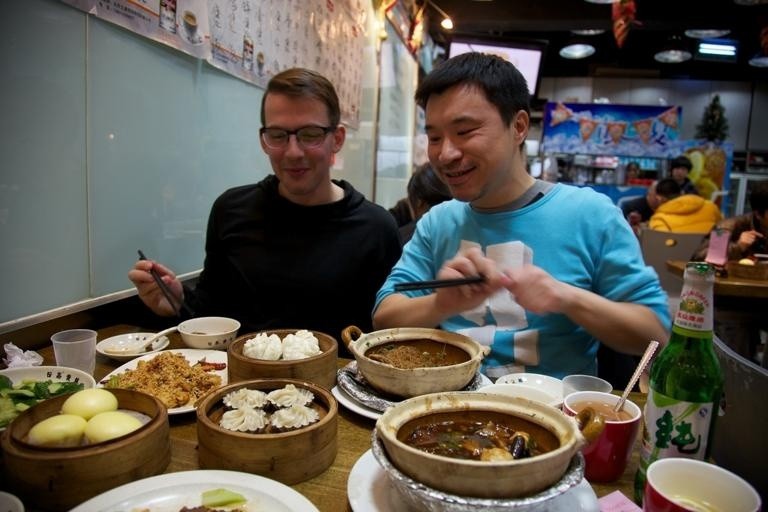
[638,227,711,297]
[705,331,766,510]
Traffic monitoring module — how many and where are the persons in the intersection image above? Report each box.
[398,162,454,249]
[620,156,768,366]
[388,197,412,227]
[370,53,675,383]
[128,69,402,361]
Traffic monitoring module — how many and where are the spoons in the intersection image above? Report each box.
[105,325,176,353]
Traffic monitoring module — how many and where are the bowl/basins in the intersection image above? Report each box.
[178,312,242,353]
[376,390,582,498]
[342,325,491,399]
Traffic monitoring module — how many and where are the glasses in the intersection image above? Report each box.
[257,124,341,150]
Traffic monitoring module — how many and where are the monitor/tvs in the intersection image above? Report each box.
[444,37,543,99]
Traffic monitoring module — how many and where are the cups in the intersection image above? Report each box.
[562,374,612,392]
[567,390,639,484]
[643,458,762,512]
[50,326,95,375]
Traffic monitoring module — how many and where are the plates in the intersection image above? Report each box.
[0,365,98,433]
[478,371,573,407]
[345,448,596,511]
[66,469,319,512]
[96,347,229,417]
[96,332,172,357]
[332,373,496,421]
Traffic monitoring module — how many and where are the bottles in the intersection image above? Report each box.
[632,259,723,508]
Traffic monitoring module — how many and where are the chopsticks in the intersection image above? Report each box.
[393,279,489,293]
[137,248,196,318]
